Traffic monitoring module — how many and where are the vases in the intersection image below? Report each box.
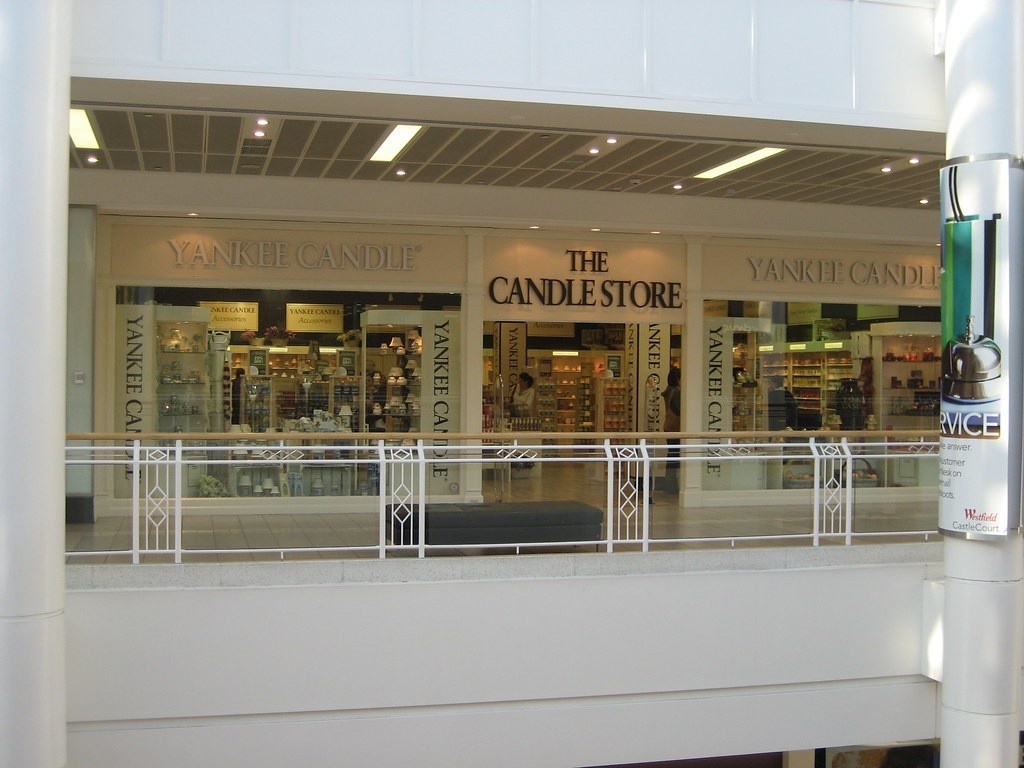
[511,468,530,479]
[271,338,288,347]
[342,340,359,349]
[248,338,265,347]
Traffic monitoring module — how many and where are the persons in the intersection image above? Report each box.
[364,374,378,403]
[769,376,797,433]
[661,369,682,494]
[509,370,536,419]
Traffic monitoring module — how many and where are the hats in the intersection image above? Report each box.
[772,375,782,384]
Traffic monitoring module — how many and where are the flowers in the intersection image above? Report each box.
[510,454,534,470]
[240,331,263,338]
[336,329,364,342]
[263,326,297,340]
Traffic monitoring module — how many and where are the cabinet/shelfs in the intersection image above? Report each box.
[480,369,630,453]
[732,331,756,442]
[871,321,940,482]
[761,339,853,410]
[157,349,209,454]
[220,349,424,496]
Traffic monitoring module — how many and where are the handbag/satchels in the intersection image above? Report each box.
[664,388,680,432]
[510,384,517,403]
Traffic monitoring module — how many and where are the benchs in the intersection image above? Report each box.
[385,500,604,554]
